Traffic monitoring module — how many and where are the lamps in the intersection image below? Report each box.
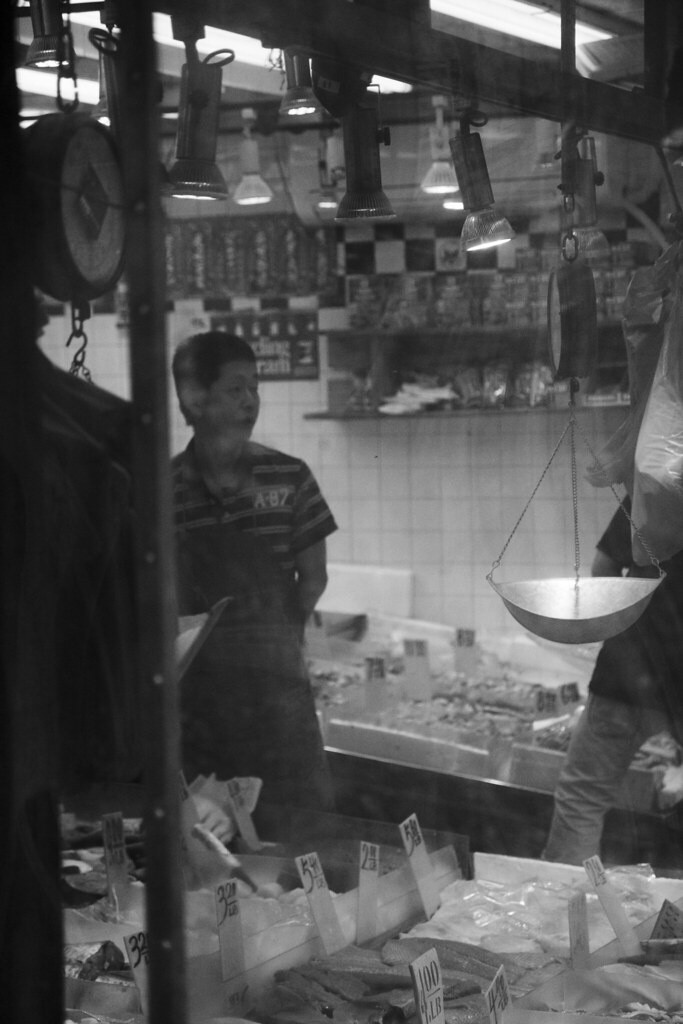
[420,94,461,196]
[235,107,274,204]
[554,136,609,266]
[24,0,72,73]
[160,14,228,202]
[277,46,333,123]
[317,130,339,209]
[448,114,516,252]
[332,82,399,223]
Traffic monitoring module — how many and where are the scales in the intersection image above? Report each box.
[485,121,668,645]
[18,11,128,381]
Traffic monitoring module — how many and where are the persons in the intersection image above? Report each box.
[172,331,336,813]
[541,495,683,863]
[0,289,146,791]
